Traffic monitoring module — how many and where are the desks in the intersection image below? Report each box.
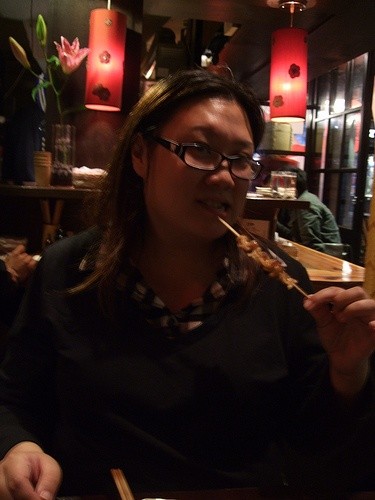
[244,192,311,245]
[0,184,110,256]
[276,236,366,293]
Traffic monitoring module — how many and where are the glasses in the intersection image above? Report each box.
[155,137,264,183]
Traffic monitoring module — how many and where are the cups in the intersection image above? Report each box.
[271,171,297,197]
[33,151,51,186]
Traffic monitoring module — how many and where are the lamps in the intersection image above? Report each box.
[186,19,242,68]
[86,0,127,111]
[268,0,309,122]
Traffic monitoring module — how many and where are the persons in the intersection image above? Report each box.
[270,163,343,254]
[0,70,375,500]
[0,237,39,315]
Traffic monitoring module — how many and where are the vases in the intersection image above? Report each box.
[50,125,76,187]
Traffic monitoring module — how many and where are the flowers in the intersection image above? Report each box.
[9,14,89,175]
